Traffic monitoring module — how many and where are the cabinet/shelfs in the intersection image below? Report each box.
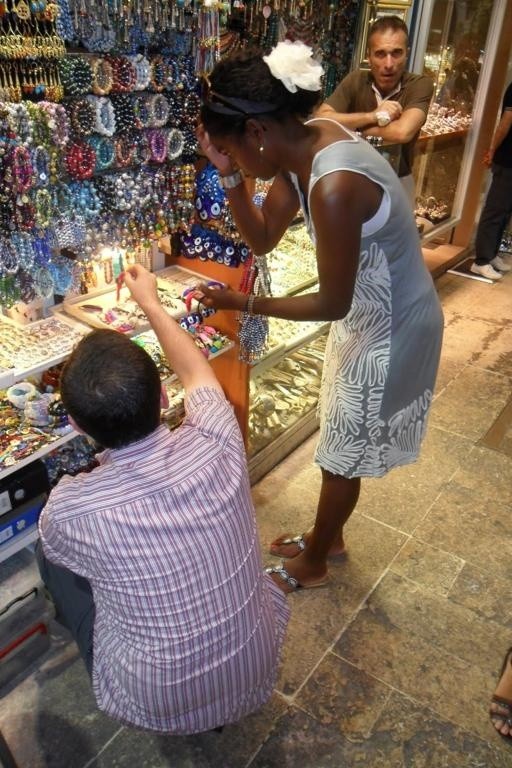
[0,264,257,690]
[362,125,469,246]
[246,173,332,486]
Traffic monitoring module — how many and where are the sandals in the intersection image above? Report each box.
[265,558,330,594]
[269,524,345,558]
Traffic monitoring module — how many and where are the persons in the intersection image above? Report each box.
[470,80,512,280]
[315,16,434,210]
[192,59,444,594]
[36,264,292,736]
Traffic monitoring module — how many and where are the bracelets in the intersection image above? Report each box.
[247,294,259,319]
[223,173,241,189]
[2,0,361,482]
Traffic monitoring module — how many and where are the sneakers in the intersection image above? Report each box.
[470,255,512,279]
